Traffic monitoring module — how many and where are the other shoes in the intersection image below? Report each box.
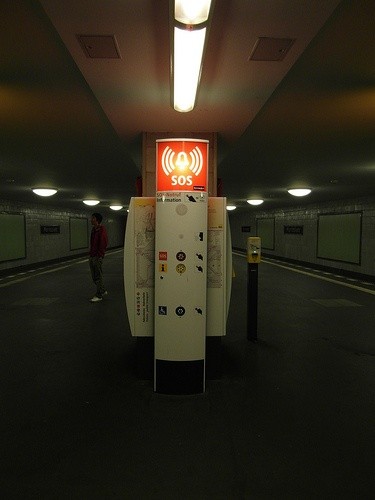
[102,291,108,296]
[90,296,102,302]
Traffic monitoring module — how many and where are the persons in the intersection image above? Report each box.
[89,212,108,301]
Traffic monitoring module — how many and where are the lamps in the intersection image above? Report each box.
[170,0,214,113]
